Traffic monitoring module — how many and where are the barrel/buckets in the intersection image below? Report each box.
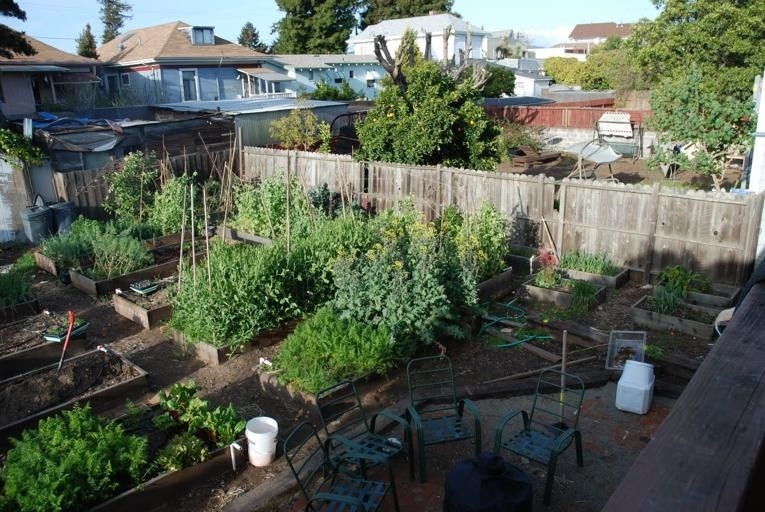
[245,416,279,468]
[616,360,657,415]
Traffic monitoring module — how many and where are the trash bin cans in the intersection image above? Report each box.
[20,202,78,247]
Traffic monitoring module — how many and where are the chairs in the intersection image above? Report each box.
[283,419,399,511]
[404,356,483,485]
[492,368,586,510]
[314,380,415,502]
